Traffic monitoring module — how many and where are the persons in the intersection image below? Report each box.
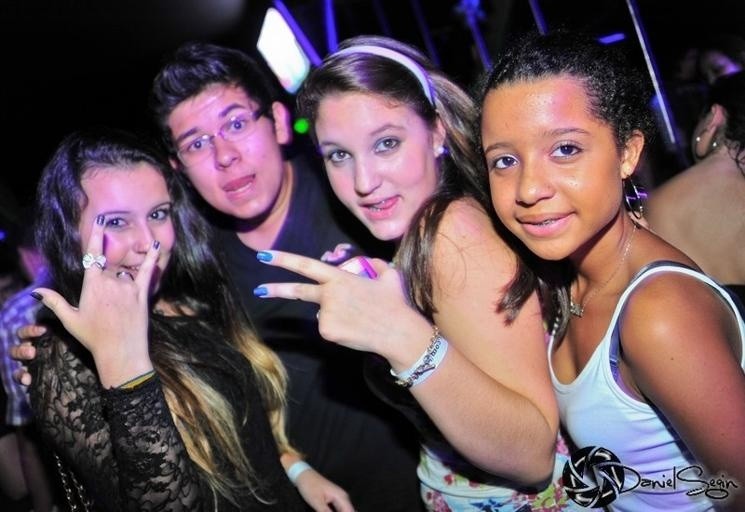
[6,38,659,512]
[249,30,563,512]
[698,32,745,85]
[0,209,57,512]
[1,239,30,508]
[637,69,745,317]
[23,125,358,512]
[477,27,745,512]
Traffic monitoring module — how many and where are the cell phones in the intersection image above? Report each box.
[337,256,378,280]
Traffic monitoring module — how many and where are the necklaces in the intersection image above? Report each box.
[569,225,636,317]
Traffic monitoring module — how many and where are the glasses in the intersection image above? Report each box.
[169,104,268,168]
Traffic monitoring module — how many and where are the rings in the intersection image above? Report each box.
[81,253,106,269]
[316,310,320,320]
[116,271,134,281]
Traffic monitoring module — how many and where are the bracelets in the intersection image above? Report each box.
[287,460,313,485]
[389,333,449,389]
[395,325,441,388]
